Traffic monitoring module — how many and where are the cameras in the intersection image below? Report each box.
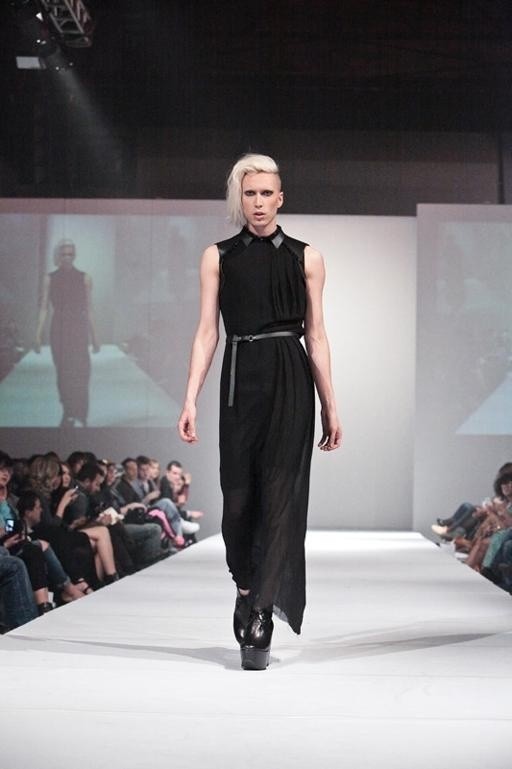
[4,517,23,535]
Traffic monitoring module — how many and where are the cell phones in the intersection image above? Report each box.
[73,483,80,496]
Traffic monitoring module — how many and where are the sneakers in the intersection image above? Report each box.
[430,516,455,542]
[37,516,201,618]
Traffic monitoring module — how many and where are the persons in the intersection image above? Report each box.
[30,235,103,430]
[424,459,511,593]
[1,314,26,380]
[174,149,343,672]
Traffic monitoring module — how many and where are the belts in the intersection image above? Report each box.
[226,329,301,409]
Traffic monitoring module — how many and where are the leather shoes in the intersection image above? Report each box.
[233,584,260,646]
[240,602,275,671]
